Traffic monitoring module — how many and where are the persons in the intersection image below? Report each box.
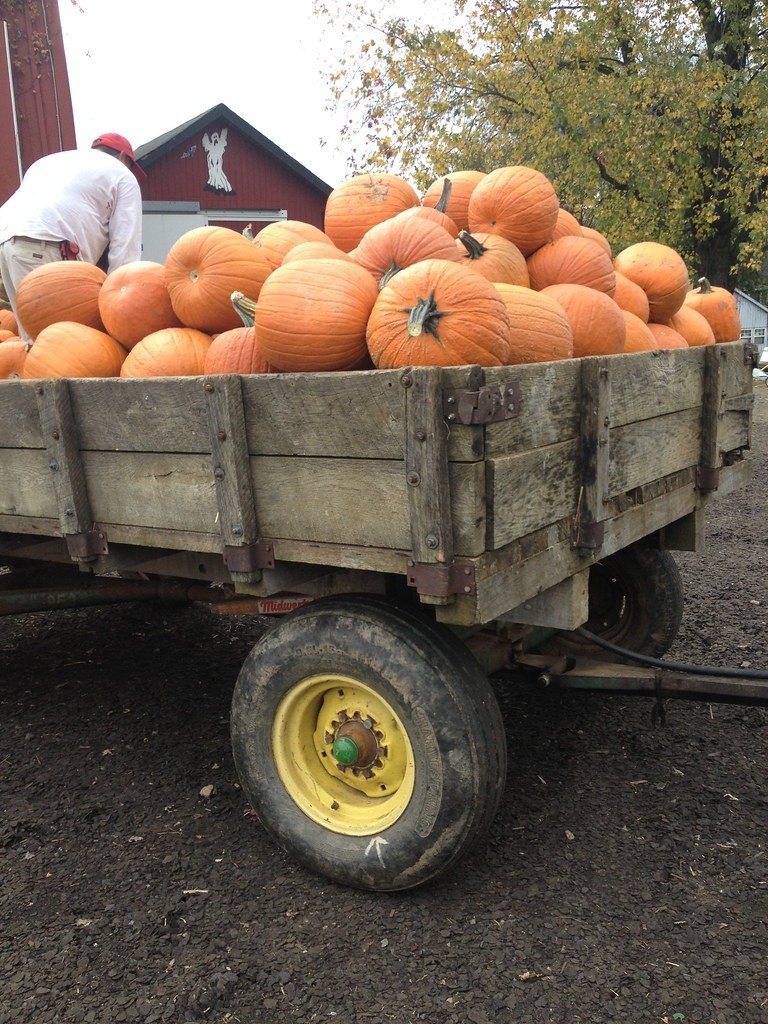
[1,133,146,346]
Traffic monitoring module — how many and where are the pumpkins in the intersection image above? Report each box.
[0,165,743,381]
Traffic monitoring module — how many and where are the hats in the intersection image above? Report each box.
[92,133,147,180]
